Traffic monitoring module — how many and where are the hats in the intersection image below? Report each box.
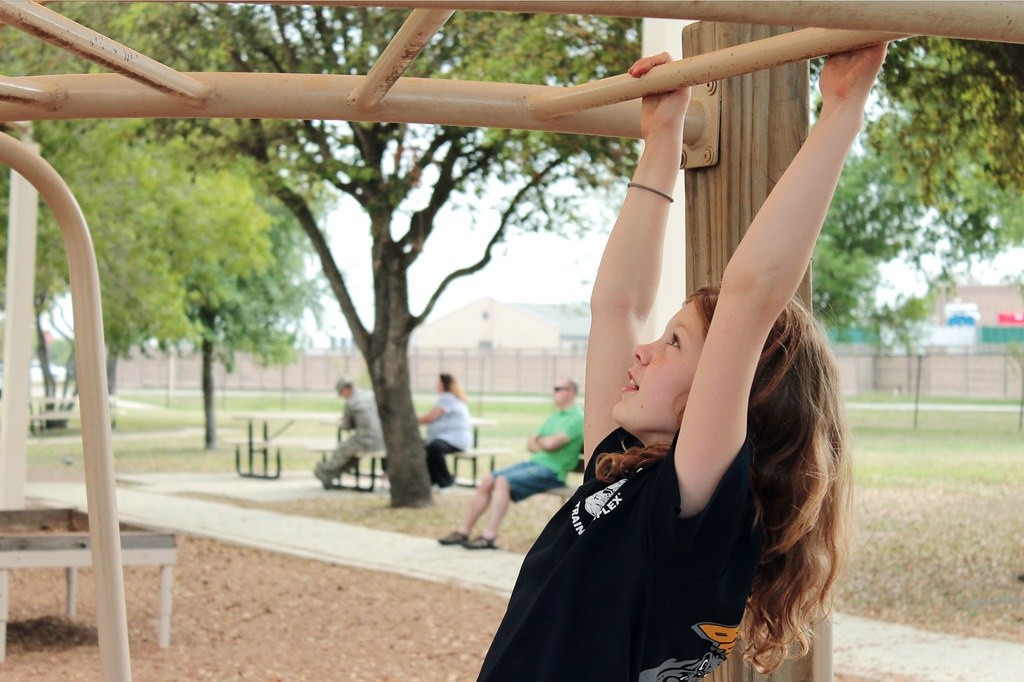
[335,377,352,397]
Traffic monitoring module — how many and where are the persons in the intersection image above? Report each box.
[315,377,385,489]
[440,377,585,548]
[417,374,474,492]
[475,43,890,682]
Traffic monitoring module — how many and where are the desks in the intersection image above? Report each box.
[221,412,497,488]
[27,395,118,428]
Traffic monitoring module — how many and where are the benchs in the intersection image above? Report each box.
[542,443,585,508]
[0,533,178,663]
[29,411,117,420]
[223,437,514,456]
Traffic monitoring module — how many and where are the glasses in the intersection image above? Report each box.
[554,387,568,392]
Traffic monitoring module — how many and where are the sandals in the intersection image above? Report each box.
[439,532,468,544]
[462,536,501,549]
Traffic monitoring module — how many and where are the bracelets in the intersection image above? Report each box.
[627,183,674,203]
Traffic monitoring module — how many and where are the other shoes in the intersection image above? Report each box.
[432,475,456,494]
[315,461,334,487]
